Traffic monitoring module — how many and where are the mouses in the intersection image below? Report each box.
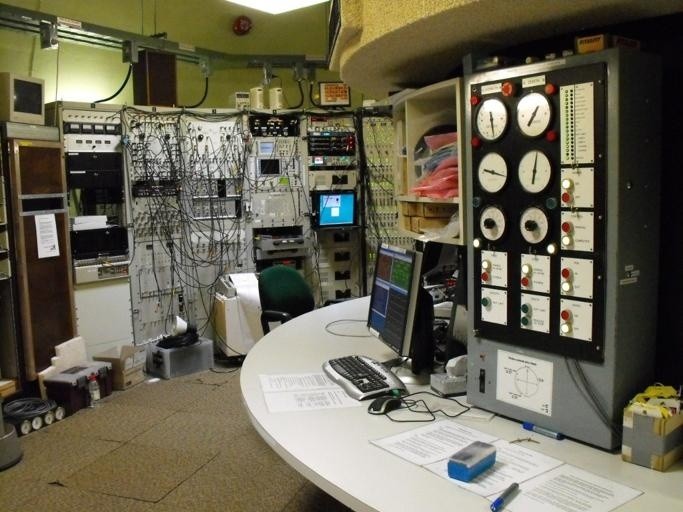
[368,395,400,414]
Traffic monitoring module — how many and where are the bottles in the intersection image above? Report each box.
[88,375,100,408]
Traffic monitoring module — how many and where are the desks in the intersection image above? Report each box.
[237,290,682,512]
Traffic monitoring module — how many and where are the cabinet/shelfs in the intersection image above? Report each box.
[391,77,465,246]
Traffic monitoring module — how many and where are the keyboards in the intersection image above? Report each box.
[322,354,405,401]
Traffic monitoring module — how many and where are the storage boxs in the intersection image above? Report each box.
[41,334,215,415]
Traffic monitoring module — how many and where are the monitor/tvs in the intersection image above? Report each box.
[318,81,351,110]
[310,189,359,227]
[367,243,435,384]
[0,71,45,126]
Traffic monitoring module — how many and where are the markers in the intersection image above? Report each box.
[490,482,519,512]
[522,421,564,440]
